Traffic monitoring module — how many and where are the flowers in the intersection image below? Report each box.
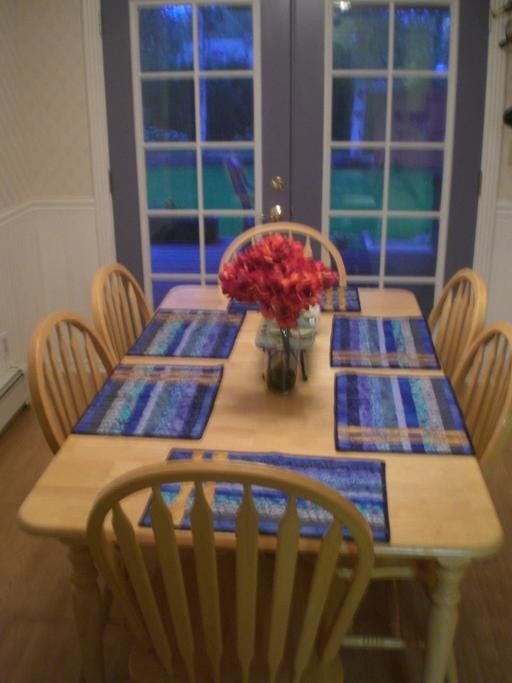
[218,232,339,365]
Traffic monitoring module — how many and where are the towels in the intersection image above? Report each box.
[330,315,441,369]
[126,308,247,358]
[72,364,223,440]
[228,286,362,312]
[334,372,476,456]
[138,449,390,542]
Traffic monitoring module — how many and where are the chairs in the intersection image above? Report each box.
[427,268,486,399]
[224,155,254,229]
[218,221,346,284]
[85,459,375,683]
[450,321,512,479]
[361,231,432,319]
[26,309,116,455]
[91,262,153,365]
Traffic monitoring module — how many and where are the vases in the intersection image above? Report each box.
[256,318,315,395]
